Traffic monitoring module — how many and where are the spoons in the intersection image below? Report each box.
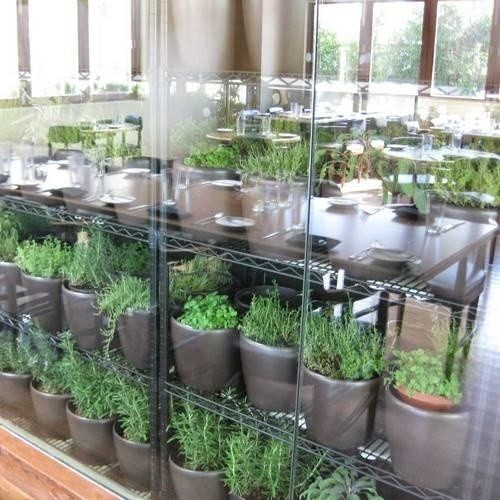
[193,211,225,224]
[261,222,304,240]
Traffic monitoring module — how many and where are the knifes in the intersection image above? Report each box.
[442,221,466,233]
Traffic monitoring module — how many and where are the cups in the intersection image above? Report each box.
[422,134,432,154]
[112,111,121,125]
[262,115,271,135]
[237,117,246,137]
[0,141,450,235]
[291,102,298,115]
[297,105,304,118]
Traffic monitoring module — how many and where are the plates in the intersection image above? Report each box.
[216,128,234,132]
[15,158,419,264]
[149,204,192,220]
[108,125,121,128]
[279,132,296,137]
[386,144,408,150]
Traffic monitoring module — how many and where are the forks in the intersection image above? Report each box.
[361,206,383,215]
